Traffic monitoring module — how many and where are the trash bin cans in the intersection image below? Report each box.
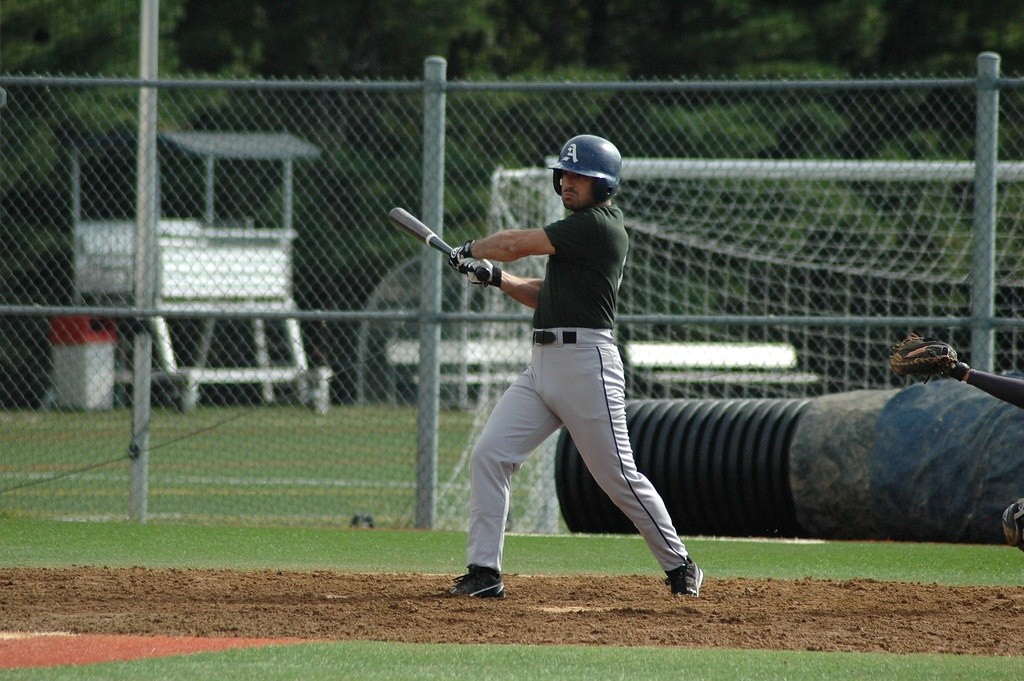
[51,312,122,410]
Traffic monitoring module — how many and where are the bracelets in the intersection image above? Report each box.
[960,368,972,384]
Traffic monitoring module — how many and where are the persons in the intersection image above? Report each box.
[447,133,704,601]
[889,333,1024,410]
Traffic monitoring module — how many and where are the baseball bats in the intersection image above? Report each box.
[386,204,494,283]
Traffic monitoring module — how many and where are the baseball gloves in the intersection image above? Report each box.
[888,331,962,379]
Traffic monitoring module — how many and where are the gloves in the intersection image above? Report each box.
[457,256,502,289]
[448,239,474,271]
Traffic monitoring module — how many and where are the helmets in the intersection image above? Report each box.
[548,134,622,205]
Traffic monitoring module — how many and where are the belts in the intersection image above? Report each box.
[531,329,578,346]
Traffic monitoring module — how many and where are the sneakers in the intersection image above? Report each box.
[445,562,504,601]
[664,553,704,598]
